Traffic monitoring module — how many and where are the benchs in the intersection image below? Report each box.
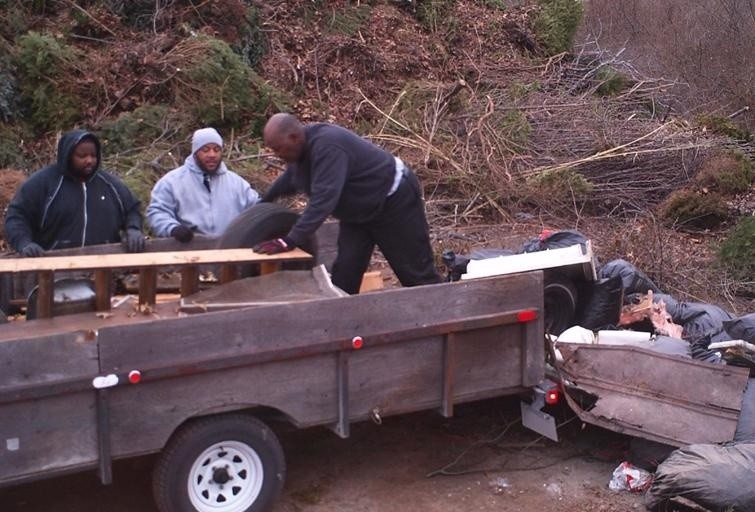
[0,236,315,322]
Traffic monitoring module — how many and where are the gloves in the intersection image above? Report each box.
[21,241,45,257]
[119,225,147,253]
[169,223,200,243]
[252,235,294,256]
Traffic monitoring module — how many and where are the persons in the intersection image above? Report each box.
[2,128,148,300]
[249,114,444,295]
[145,128,269,280]
